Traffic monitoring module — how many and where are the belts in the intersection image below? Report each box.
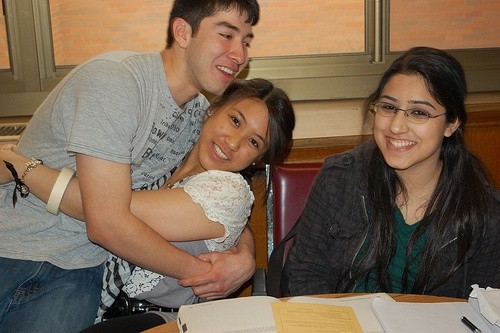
[144,305,179,313]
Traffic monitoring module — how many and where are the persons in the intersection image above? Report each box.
[284,47,500,299]
[0,80,295,333]
[0,0,256,333]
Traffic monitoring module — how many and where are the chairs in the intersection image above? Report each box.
[265,162,324,270]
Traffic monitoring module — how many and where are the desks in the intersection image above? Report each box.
[142,292,468,333]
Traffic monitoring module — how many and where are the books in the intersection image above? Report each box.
[177,291,500,333]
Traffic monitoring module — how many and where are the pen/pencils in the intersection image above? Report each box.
[460,315,482,333]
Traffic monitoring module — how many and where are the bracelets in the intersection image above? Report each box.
[46,167,76,214]
[4,157,43,205]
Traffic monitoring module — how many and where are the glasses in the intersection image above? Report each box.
[368,100,448,123]
